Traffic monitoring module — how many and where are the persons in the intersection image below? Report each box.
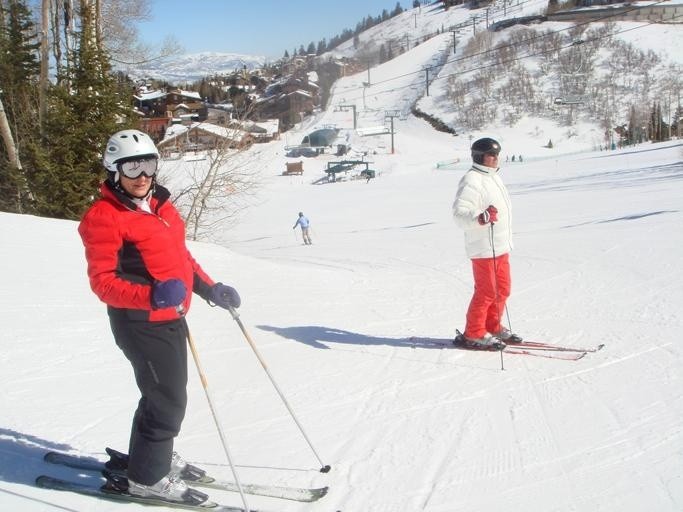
[292,211,312,245]
[75,130,241,501]
[451,137,522,348]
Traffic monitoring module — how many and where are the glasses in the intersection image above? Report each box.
[117,157,159,180]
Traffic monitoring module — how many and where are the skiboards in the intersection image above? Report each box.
[36,451,328,511]
[410,337,604,360]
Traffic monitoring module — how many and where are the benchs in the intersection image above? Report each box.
[285,161,304,176]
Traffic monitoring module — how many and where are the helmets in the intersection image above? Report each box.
[103,129,161,172]
[471,138,501,163]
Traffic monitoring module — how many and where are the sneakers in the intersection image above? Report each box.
[493,327,512,340]
[463,332,498,346]
[172,452,186,475]
[128,473,188,501]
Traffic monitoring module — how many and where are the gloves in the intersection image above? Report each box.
[209,282,241,308]
[478,207,497,226]
[150,278,188,312]
